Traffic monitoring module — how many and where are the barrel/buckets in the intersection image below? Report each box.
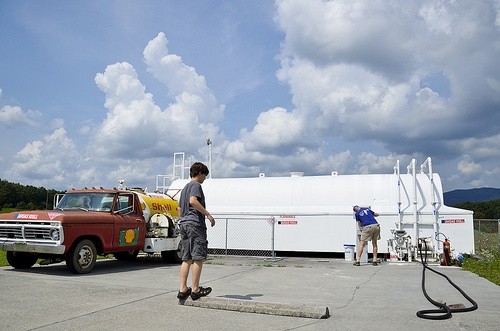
[344,244,356,262]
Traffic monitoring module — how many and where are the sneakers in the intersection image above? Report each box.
[177,287,192,300]
[190,286,212,301]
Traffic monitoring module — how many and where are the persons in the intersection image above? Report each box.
[177,162,215,300]
[353,205,380,266]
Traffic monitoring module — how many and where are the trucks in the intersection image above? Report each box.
[0,185,181,273]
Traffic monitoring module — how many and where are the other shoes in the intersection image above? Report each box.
[372,262,377,266]
[353,262,360,266]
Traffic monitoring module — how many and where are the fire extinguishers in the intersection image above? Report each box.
[440,238,450,266]
